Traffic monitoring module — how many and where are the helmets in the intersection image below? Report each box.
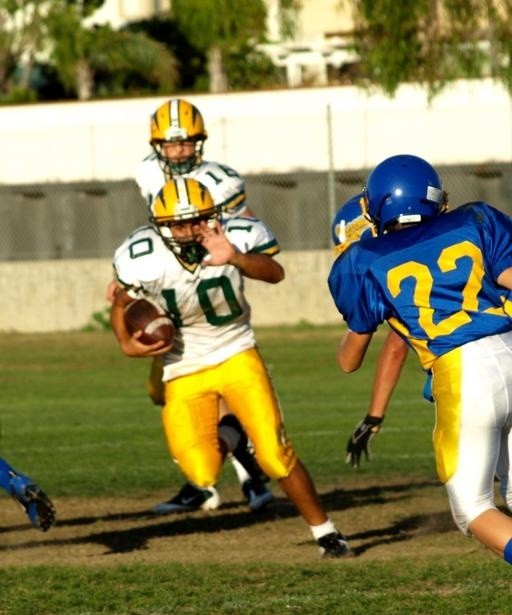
[149,100,208,176]
[361,155,446,238]
[147,176,222,265]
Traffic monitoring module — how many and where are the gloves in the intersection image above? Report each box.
[344,412,384,470]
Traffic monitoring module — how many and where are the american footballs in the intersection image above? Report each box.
[123,297,175,349]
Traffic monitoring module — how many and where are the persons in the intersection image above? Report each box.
[329,153,512,564]
[0,460,55,532]
[331,194,434,470]
[110,176,352,559]
[133,98,272,512]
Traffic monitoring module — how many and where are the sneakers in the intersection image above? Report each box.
[314,528,355,560]
[241,477,272,511]
[152,483,222,514]
[217,414,269,483]
[10,472,56,533]
